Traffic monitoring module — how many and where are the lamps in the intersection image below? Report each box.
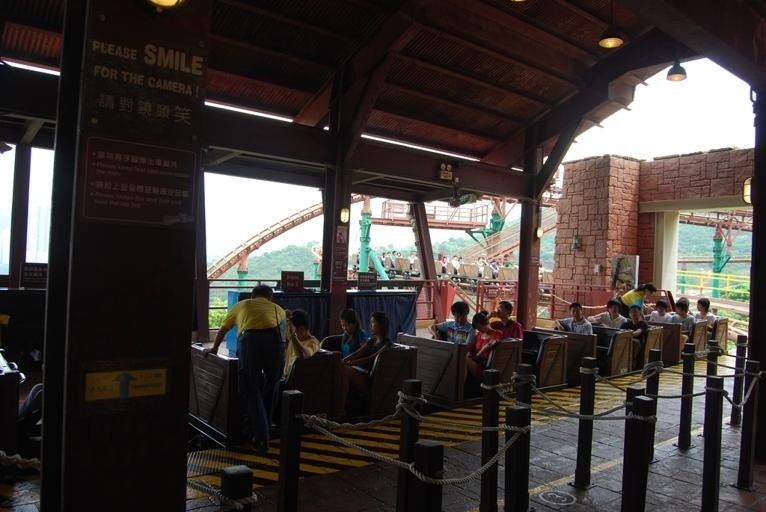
[668,48,686,81]
[597,0,624,49]
[339,192,350,224]
[742,176,752,205]
[536,206,544,239]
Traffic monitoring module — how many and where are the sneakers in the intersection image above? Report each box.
[252,433,272,451]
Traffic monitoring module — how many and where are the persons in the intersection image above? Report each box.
[343,311,391,371]
[587,282,717,369]
[381,250,418,271]
[203,285,287,455]
[284,308,320,381]
[428,301,524,385]
[337,308,371,360]
[437,253,464,274]
[476,254,514,279]
[554,302,593,335]
